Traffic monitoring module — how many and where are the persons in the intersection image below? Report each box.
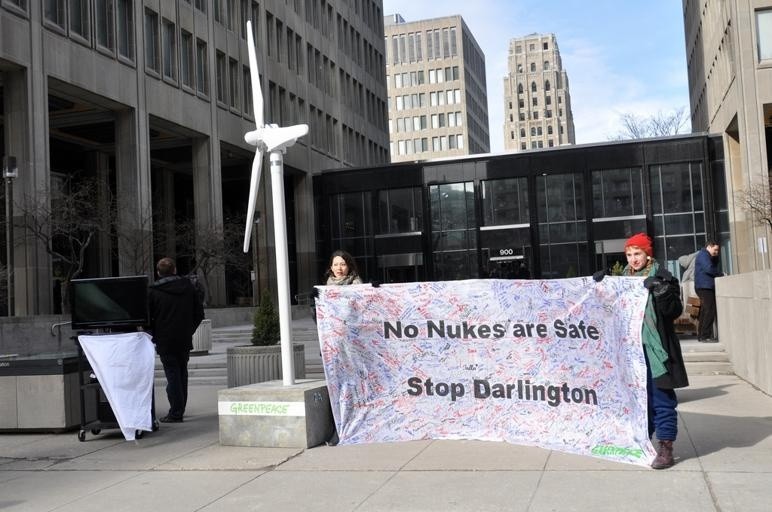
[309,249,380,447]
[148,257,205,422]
[694,239,727,342]
[594,233,689,469]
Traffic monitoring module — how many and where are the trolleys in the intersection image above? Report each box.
[50,275,159,441]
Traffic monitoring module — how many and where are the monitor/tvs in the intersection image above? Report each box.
[67,275,149,329]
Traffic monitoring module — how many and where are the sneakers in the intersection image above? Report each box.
[160,416,182,424]
[697,336,717,343]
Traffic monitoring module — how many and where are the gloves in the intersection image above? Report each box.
[369,279,382,288]
[592,270,609,282]
[643,276,663,290]
[309,288,319,299]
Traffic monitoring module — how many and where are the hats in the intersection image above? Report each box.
[624,233,653,257]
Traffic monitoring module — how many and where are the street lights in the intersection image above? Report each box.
[2,156,19,316]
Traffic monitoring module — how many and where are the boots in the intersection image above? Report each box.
[652,440,673,469]
